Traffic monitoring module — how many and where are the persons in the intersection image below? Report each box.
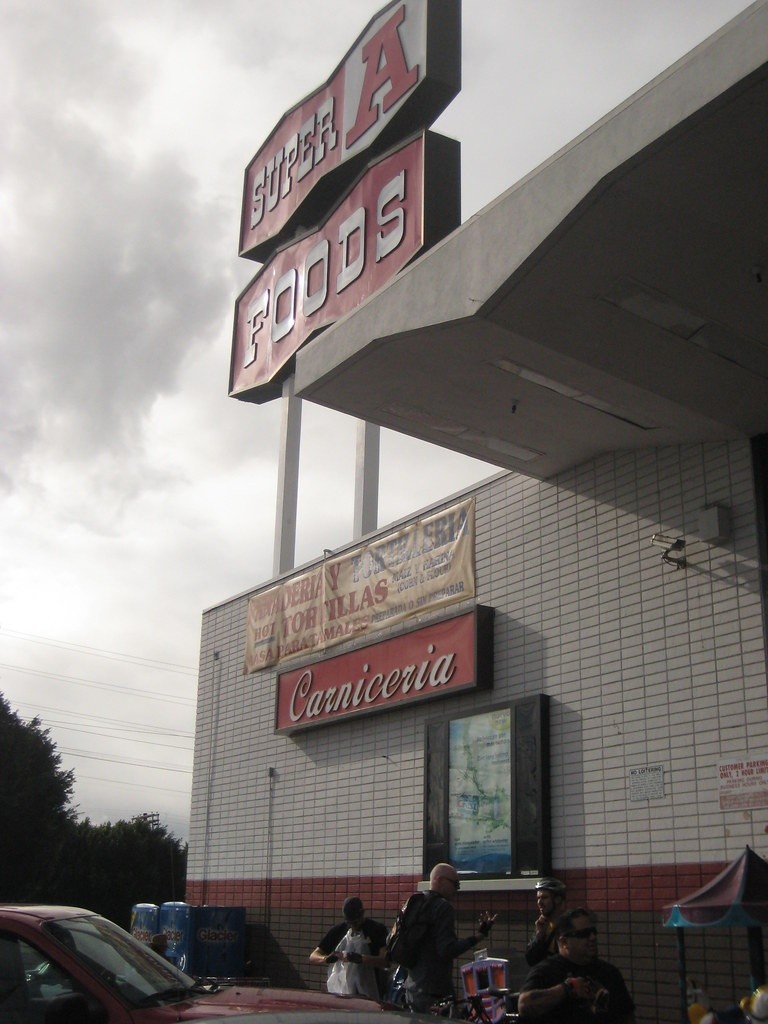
[517,876,635,1024]
[309,896,390,1001]
[148,935,171,964]
[386,863,498,1013]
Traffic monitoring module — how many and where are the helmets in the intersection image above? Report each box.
[531,876,576,900]
[337,896,365,918]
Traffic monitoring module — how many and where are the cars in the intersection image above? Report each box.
[1,905,386,1024]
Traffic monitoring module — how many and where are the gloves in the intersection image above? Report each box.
[475,920,495,938]
[327,955,340,964]
[345,953,364,967]
[565,975,597,1002]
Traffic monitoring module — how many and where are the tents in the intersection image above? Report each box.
[662,845,768,1024]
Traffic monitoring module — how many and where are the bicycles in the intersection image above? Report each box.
[430,987,520,1024]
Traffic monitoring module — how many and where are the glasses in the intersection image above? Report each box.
[442,876,464,885]
[559,924,596,940]
